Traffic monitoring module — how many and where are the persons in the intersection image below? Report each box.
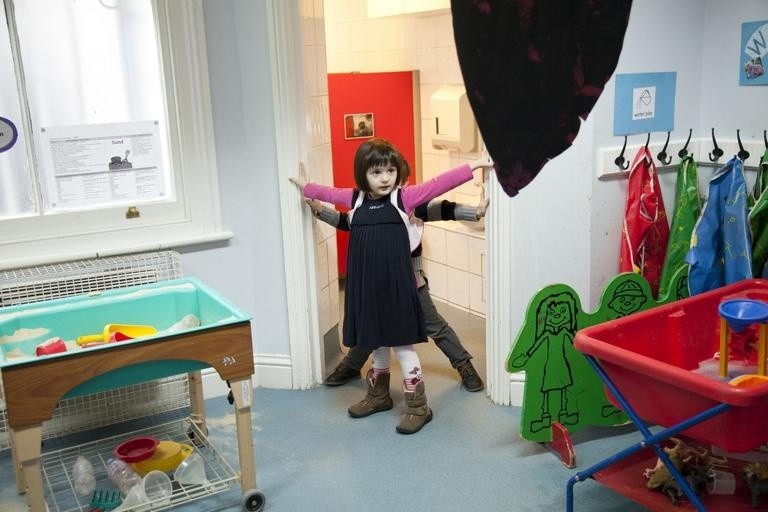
[288,137,495,434]
[304,159,491,392]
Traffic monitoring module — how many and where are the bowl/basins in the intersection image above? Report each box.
[112,436,161,464]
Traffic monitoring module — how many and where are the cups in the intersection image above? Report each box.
[706,468,737,496]
[36,337,68,357]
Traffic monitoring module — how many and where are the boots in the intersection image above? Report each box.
[396,378,432,432]
[348,368,392,417]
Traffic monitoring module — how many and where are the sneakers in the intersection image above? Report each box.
[326,356,361,385]
[457,362,484,392]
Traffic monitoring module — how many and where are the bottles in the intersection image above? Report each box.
[106,457,143,496]
[72,455,97,496]
